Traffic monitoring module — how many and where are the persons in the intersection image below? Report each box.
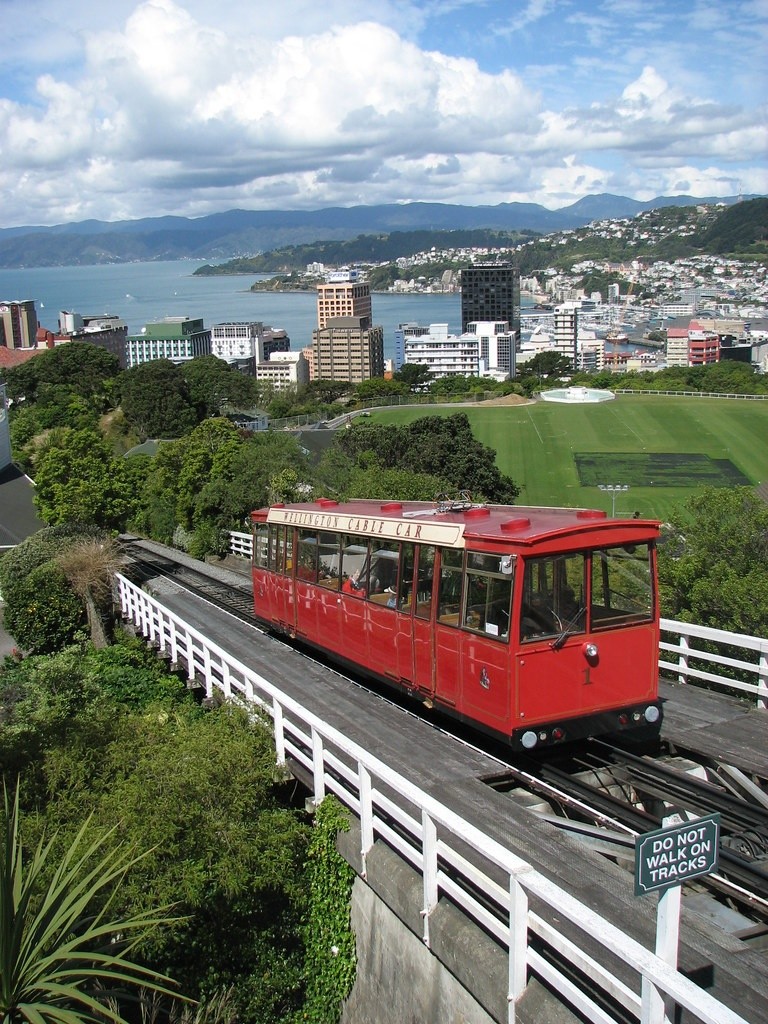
[262,533,584,639]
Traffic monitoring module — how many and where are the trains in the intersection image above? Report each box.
[248,495,667,762]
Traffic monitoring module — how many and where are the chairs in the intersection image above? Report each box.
[257,557,578,641]
